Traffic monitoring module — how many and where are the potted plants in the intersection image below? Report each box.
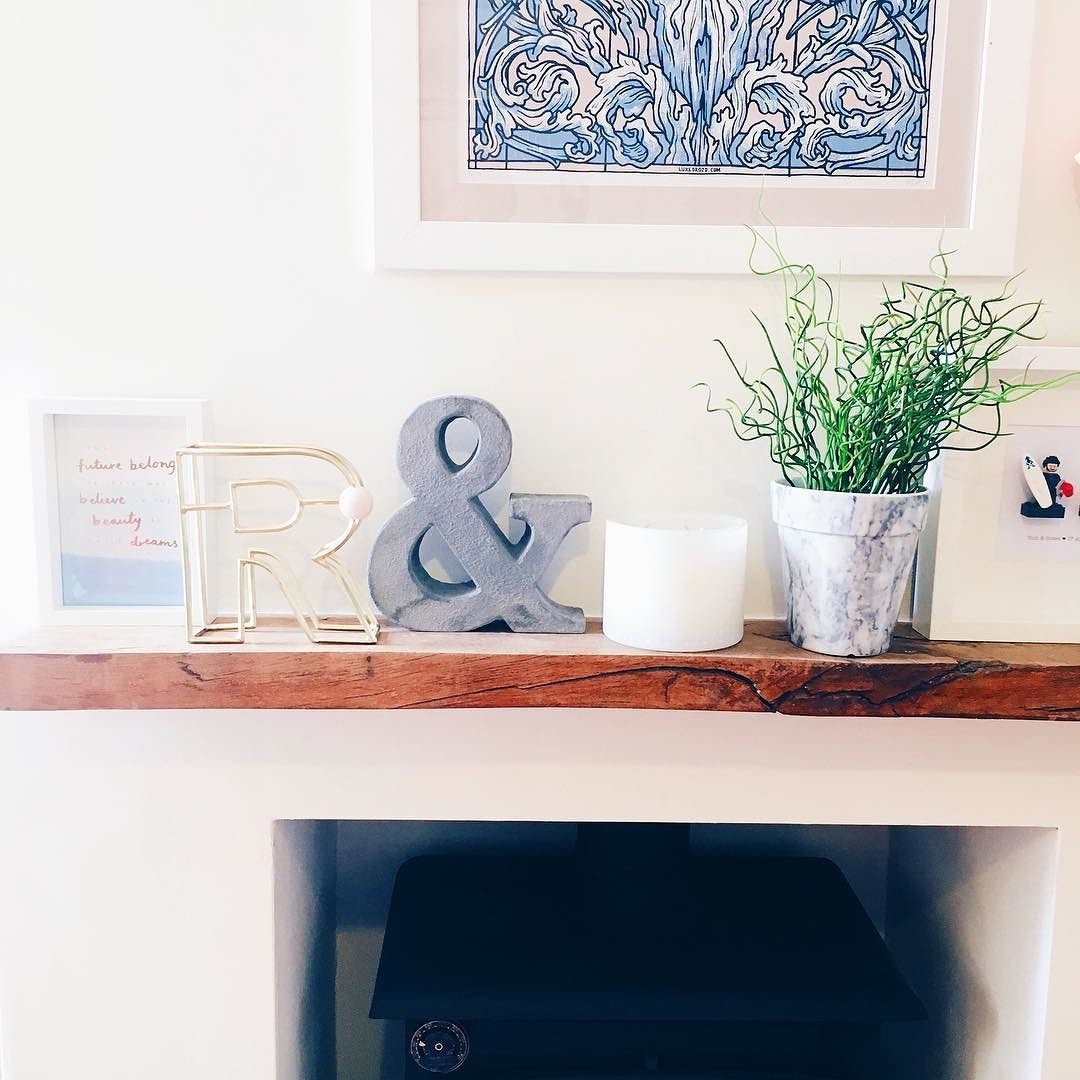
[690,178,1080,658]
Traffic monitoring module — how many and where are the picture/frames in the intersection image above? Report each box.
[912,344,1080,645]
[365,0,1036,283]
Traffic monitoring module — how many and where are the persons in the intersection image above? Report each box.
[1040,456,1060,506]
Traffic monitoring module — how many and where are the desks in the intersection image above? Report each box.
[1,614,1080,1080]
